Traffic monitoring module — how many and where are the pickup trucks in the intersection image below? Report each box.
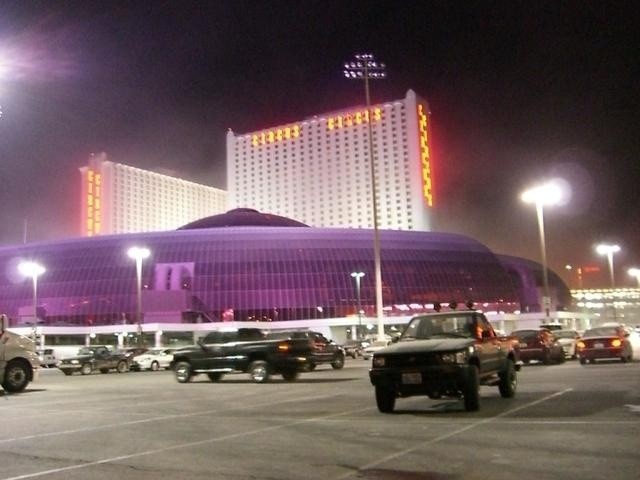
[369,309,525,414]
[59,345,132,375]
[170,329,313,383]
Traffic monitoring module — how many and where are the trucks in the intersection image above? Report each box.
[0,314,39,393]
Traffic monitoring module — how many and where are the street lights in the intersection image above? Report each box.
[350,270,365,338]
[343,51,386,339]
[19,261,45,344]
[518,180,562,321]
[128,248,149,349]
[596,244,620,292]
[627,267,640,288]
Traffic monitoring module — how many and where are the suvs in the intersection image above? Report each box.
[302,331,344,370]
[539,323,565,332]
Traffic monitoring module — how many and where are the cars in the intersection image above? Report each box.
[361,340,392,359]
[35,349,58,369]
[550,328,580,359]
[114,346,149,363]
[578,324,634,363]
[131,347,178,371]
[338,341,370,358]
[510,328,565,364]
